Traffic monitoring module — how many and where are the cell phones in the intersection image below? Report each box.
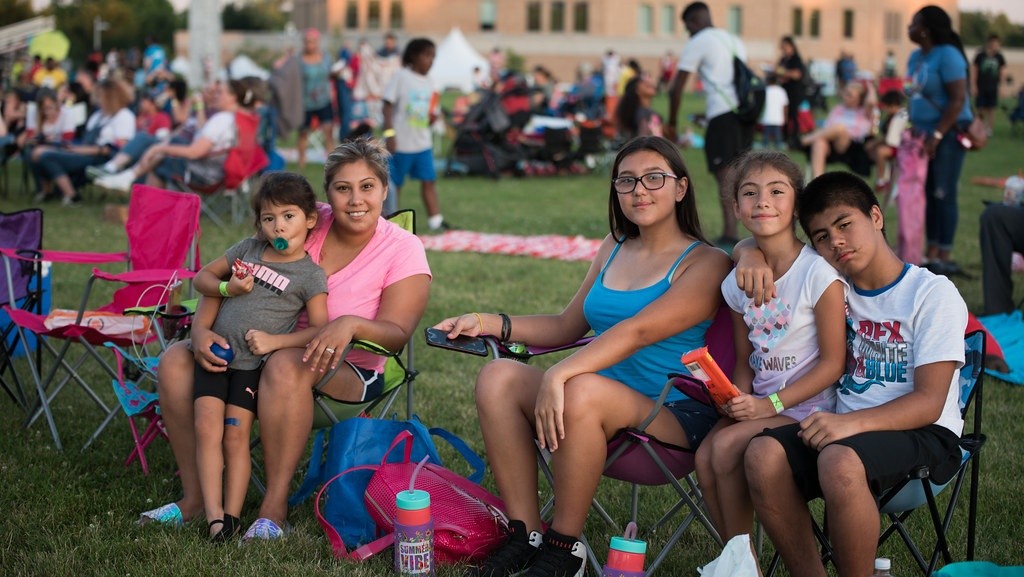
[426,329,491,356]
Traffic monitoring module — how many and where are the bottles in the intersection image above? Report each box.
[872,557,894,577]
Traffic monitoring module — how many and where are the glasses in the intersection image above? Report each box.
[612,172,678,194]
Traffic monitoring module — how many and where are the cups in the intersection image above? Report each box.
[394,488,435,577]
[1003,175,1024,208]
[603,536,647,577]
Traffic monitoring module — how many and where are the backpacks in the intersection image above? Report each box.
[315,430,549,566]
[708,27,765,126]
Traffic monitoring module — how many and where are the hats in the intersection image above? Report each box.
[304,28,320,38]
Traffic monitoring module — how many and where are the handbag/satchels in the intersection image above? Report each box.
[956,114,988,150]
[801,66,819,96]
[324,419,444,548]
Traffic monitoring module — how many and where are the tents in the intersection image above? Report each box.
[427,24,490,94]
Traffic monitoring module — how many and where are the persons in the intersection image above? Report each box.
[135,136,433,540]
[697,155,970,577]
[686,37,910,185]
[0,44,274,224]
[454,45,677,178]
[981,151,1024,315]
[192,173,328,543]
[430,137,733,577]
[904,6,973,270]
[275,29,402,165]
[382,39,446,229]
[974,32,1008,135]
[667,0,765,251]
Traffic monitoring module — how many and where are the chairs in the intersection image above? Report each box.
[175,109,271,235]
[0,183,986,577]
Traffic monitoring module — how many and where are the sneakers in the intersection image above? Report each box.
[477,520,545,577]
[524,529,590,577]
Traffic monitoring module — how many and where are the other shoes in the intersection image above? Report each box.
[874,181,889,192]
[104,205,128,224]
[94,175,130,194]
[86,166,112,180]
[987,128,992,137]
[35,193,55,203]
[62,193,83,206]
[209,513,241,547]
[788,135,808,150]
[432,221,461,234]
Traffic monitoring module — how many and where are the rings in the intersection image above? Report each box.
[324,347,334,353]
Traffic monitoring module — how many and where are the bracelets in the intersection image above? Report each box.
[217,279,231,297]
[382,130,394,142]
[476,311,485,336]
[931,128,947,143]
[769,392,785,415]
[500,312,512,344]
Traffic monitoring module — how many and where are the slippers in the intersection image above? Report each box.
[241,518,290,545]
[135,503,192,527]
